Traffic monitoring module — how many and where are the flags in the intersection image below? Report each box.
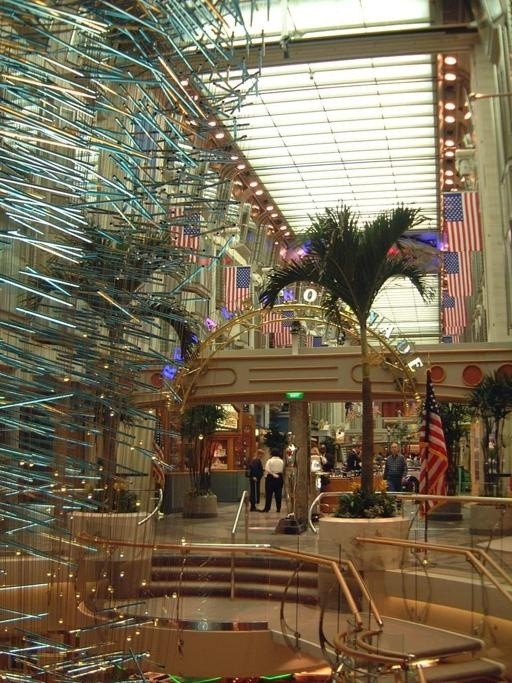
[414,365,450,520]
[432,233,474,344]
[224,266,251,312]
[261,290,323,348]
[442,191,484,254]
[166,205,200,264]
[149,417,166,486]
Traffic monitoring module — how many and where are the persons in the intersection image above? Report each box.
[345,448,362,471]
[308,446,329,515]
[248,448,266,512]
[319,443,336,473]
[382,442,409,491]
[259,446,285,512]
